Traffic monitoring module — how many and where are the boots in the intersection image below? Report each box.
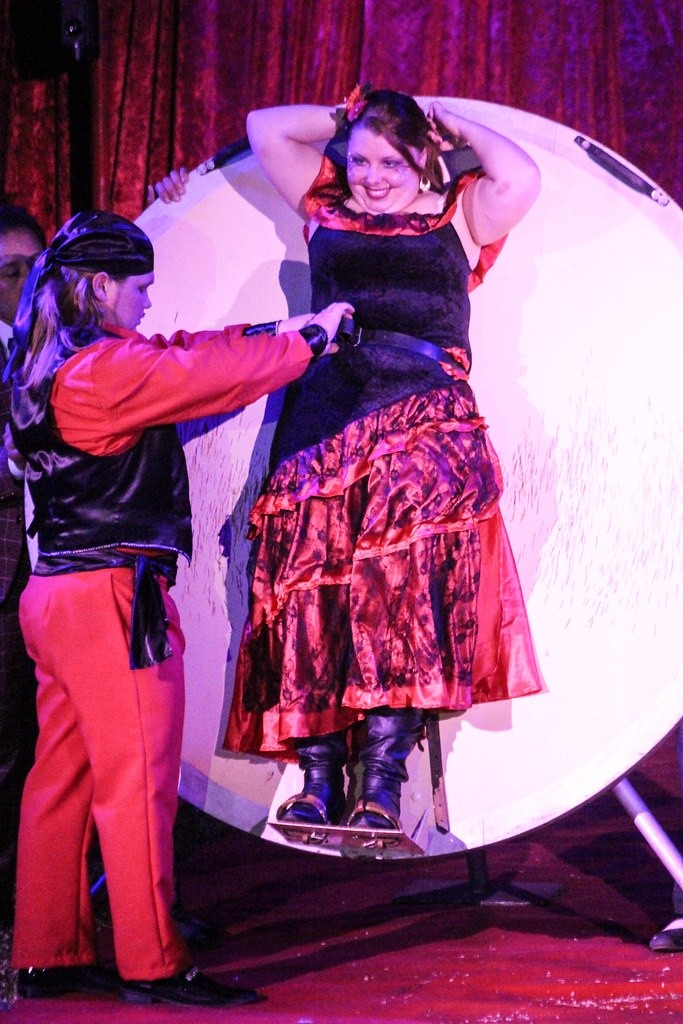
[284,727,348,826]
[349,708,424,830]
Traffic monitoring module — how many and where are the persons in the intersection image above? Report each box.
[221,83,546,833]
[0,168,355,1012]
[649,881,683,952]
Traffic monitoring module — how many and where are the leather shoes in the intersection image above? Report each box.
[16,960,123,998]
[118,958,260,1007]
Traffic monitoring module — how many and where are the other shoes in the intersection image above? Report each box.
[650,914,683,951]
